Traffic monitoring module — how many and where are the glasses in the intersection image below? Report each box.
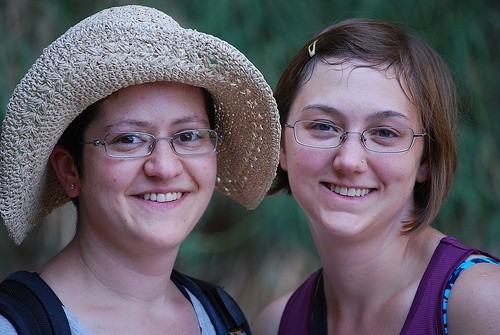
[85,127,219,158]
[285,119,426,153]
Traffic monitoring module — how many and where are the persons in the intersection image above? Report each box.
[251,17,500,335]
[0,5,282,335]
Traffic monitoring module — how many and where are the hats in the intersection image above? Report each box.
[0,5,281,246]
